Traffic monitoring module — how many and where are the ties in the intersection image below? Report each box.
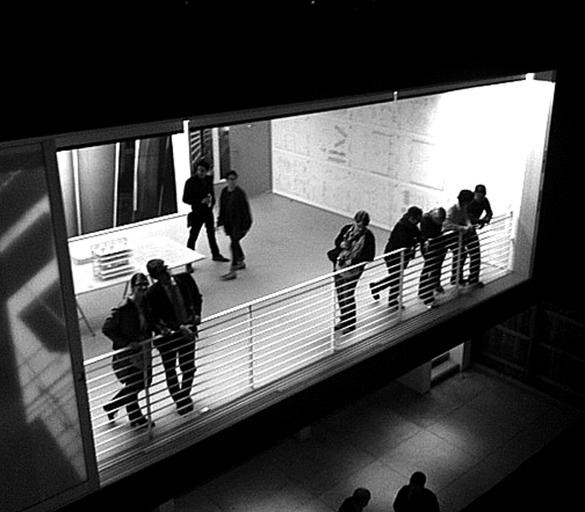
[169,284,184,326]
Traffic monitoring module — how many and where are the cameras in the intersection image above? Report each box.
[339,256,349,266]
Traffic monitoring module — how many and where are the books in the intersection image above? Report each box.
[91,240,134,282]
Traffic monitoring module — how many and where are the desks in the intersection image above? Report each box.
[67,232,207,338]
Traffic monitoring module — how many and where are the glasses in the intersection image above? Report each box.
[135,282,150,287]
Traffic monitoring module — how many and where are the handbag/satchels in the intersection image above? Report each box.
[327,248,341,264]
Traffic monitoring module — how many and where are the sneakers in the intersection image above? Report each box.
[370,282,379,300]
[335,322,356,334]
[213,255,245,281]
[187,266,194,273]
[389,301,404,311]
[177,398,193,415]
[102,404,117,425]
[130,418,155,428]
[435,278,479,293]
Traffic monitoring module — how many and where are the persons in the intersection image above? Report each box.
[393,470,440,511]
[333,210,377,334]
[370,205,431,310]
[339,487,370,511]
[441,190,482,287]
[464,184,493,285]
[144,259,203,416]
[214,171,253,280]
[101,272,174,428]
[181,161,231,263]
[418,206,446,306]
